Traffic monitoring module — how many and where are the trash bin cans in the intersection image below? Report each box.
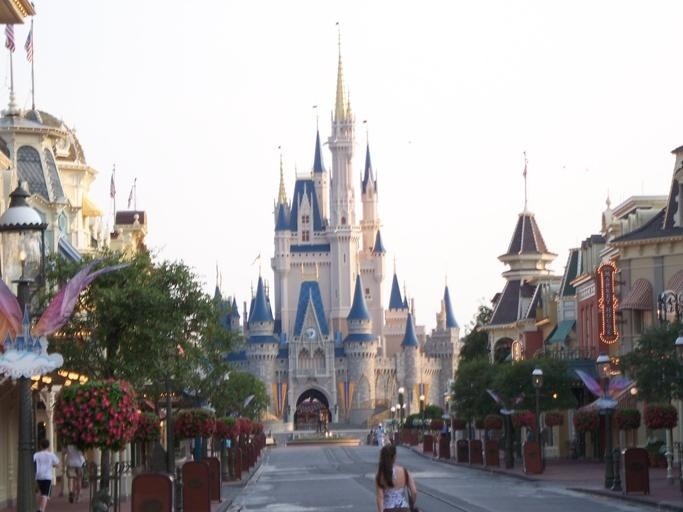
[456,439,469,462]
[621,447,649,496]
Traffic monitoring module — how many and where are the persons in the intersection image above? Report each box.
[373,443,418,511]
[31,438,61,511]
[320,408,332,437]
[62,444,87,504]
[317,409,323,433]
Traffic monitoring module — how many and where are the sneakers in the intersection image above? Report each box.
[69,492,73,503]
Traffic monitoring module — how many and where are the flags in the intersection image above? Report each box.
[2,23,15,54]
[126,182,137,209]
[108,173,116,198]
[22,19,32,64]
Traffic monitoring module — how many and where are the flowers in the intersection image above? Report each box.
[432,401,678,431]
[49,379,263,447]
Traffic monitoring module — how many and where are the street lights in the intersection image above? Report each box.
[1,180,55,512]
[532,362,546,472]
[676,334,683,346]
[390,383,427,435]
[596,350,621,489]
